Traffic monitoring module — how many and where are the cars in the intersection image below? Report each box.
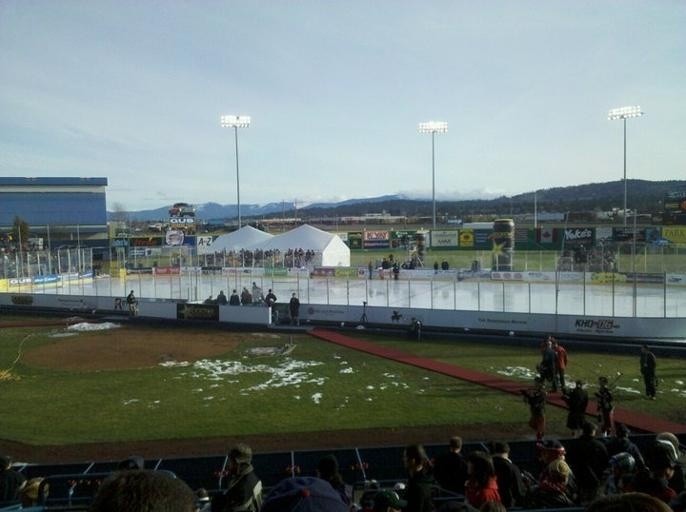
[146,217,224,234]
[166,202,196,217]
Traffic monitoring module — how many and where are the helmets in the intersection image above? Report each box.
[230,444,252,466]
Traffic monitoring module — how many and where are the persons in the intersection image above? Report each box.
[365,233,450,282]
[172,245,315,268]
[122,281,302,327]
[411,318,421,342]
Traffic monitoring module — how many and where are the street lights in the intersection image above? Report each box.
[417,120,452,230]
[607,104,645,230]
[218,115,253,231]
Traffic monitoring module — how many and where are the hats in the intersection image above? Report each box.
[261,475,350,512]
[359,482,408,512]
[609,451,635,472]
[536,439,566,454]
